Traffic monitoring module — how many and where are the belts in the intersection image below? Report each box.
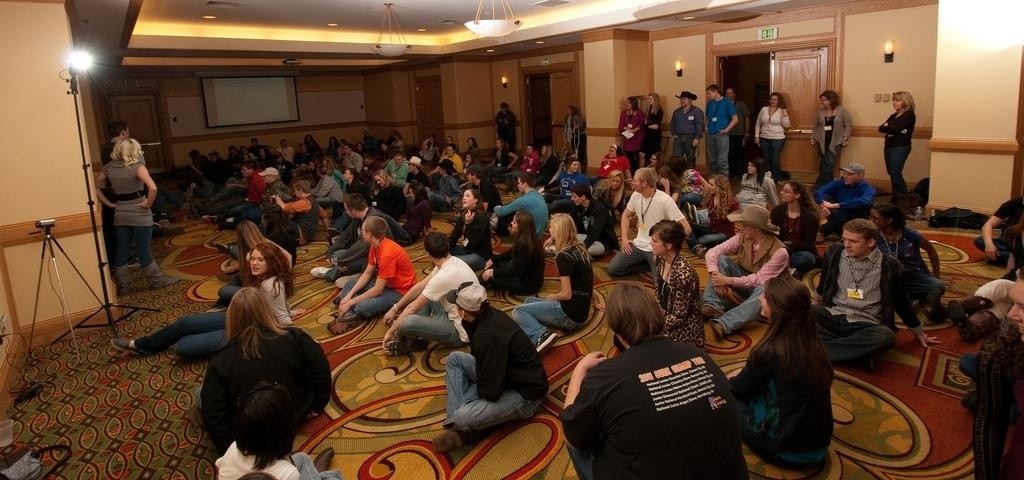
[116,190,145,201]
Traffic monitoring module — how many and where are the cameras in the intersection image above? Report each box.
[36,219,57,230]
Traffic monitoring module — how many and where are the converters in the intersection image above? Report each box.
[16,384,40,400]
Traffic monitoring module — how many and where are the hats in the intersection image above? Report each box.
[469,165,482,177]
[727,205,780,235]
[447,282,488,312]
[258,167,279,177]
[676,92,697,99]
[838,163,865,175]
[439,159,457,173]
[406,156,422,168]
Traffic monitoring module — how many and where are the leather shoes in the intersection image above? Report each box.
[313,447,334,474]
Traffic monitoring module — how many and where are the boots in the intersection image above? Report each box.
[158,219,184,235]
[899,194,922,217]
[925,288,1000,340]
[140,259,179,288]
[113,263,135,295]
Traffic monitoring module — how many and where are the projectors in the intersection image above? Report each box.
[282,58,303,68]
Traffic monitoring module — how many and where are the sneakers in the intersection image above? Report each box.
[326,313,363,334]
[310,267,332,279]
[433,425,474,452]
[700,306,724,341]
[384,339,428,356]
[335,272,362,289]
[202,215,215,224]
[111,338,140,355]
[536,330,559,353]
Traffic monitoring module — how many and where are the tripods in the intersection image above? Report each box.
[24,234,112,365]
[48,104,163,345]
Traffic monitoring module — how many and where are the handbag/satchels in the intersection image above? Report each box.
[928,207,989,228]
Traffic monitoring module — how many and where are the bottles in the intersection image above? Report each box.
[914,206,922,224]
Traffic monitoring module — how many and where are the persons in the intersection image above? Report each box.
[93,85,1024,480]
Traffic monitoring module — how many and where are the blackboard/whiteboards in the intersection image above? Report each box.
[166,89,366,138]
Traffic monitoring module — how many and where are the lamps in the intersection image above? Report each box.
[501,74,507,88]
[463,1,522,40]
[368,2,414,59]
[48,46,162,350]
[883,38,893,64]
[674,59,683,77]
